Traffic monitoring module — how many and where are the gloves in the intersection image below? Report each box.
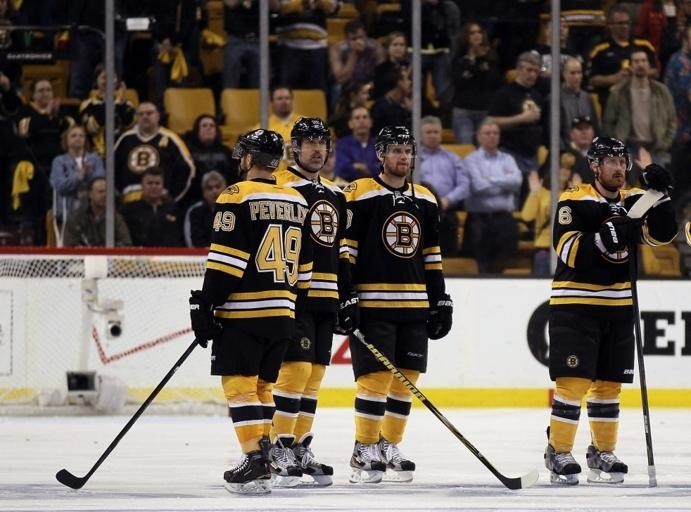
[429,294,452,339]
[338,290,360,330]
[598,216,644,253]
[189,290,224,349]
[639,164,674,208]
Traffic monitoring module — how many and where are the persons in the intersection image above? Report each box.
[343,122,453,470]
[271,118,351,478]
[0,0,691,279]
[546,135,679,474]
[189,130,314,484]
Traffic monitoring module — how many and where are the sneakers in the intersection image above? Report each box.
[292,434,332,475]
[224,440,271,483]
[544,445,581,474]
[587,445,628,473]
[350,440,386,472]
[267,435,302,477]
[377,437,415,470]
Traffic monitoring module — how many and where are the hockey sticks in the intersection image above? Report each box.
[627,188,665,488]
[55,338,198,489]
[350,327,540,490]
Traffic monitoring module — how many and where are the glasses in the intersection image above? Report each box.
[611,20,632,26]
[572,115,591,130]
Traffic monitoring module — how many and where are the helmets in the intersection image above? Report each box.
[233,129,284,169]
[290,117,331,152]
[375,126,418,159]
[586,137,633,171]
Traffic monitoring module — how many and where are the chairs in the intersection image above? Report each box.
[86,85,328,147]
